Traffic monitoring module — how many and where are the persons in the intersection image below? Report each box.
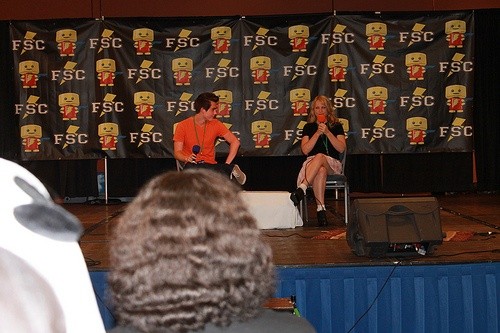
[173,92,246,186]
[102,168,316,333]
[290,96,346,228]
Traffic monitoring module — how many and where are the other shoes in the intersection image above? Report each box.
[289,188,304,206]
[230,163,246,185]
[317,210,328,227]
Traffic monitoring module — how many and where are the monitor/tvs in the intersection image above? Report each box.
[21,159,99,204]
[311,153,382,198]
[106,158,177,203]
[382,151,473,197]
[215,155,308,191]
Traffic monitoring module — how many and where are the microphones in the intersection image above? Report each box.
[192,145,200,164]
[317,113,326,137]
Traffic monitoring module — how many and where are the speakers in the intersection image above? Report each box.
[346,197,442,258]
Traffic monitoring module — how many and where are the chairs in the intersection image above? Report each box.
[298,144,352,225]
[173,123,187,175]
